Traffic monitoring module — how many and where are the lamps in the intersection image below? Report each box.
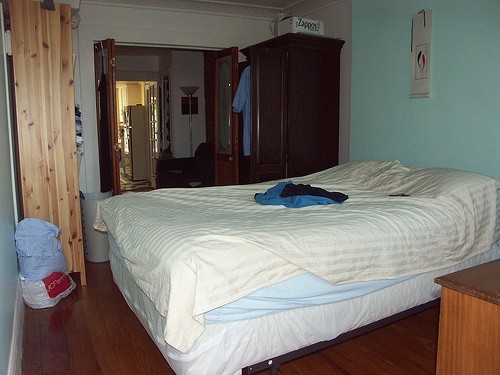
[180,86,200,158]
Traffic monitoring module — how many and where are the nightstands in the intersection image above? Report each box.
[434,259,499,375]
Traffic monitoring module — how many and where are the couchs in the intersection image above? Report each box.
[158,142,215,188]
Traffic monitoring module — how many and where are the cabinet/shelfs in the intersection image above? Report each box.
[236,33,345,184]
[7,1,86,286]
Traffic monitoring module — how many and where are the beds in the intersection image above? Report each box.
[91,161,500,375]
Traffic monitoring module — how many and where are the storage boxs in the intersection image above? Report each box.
[278,16,324,36]
